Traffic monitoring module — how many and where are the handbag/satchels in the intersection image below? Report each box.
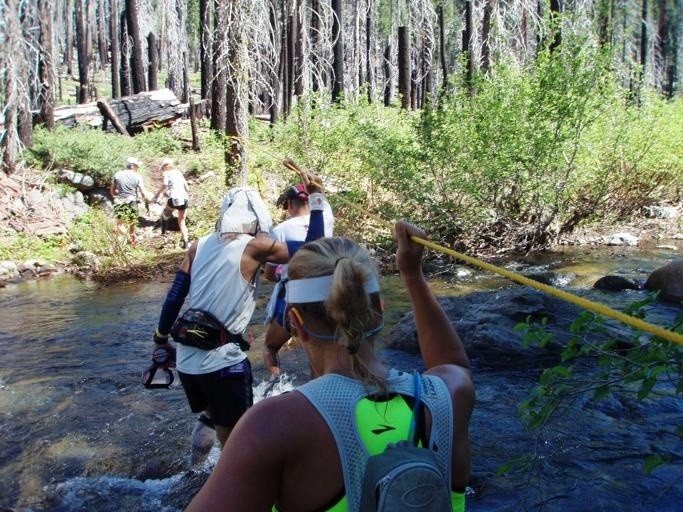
[171,192,185,207]
[359,441,451,512]
[168,308,250,351]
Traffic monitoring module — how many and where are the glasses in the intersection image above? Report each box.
[141,348,174,389]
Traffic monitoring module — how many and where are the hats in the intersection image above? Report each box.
[159,159,173,171]
[283,184,308,210]
[127,158,142,168]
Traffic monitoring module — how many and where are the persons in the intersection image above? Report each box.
[263,183,335,375]
[109,157,147,249]
[183,223,477,512]
[153,170,325,464]
[152,161,189,250]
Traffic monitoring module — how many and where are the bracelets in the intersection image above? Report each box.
[154,335,168,345]
[308,192,323,211]
[155,328,168,339]
